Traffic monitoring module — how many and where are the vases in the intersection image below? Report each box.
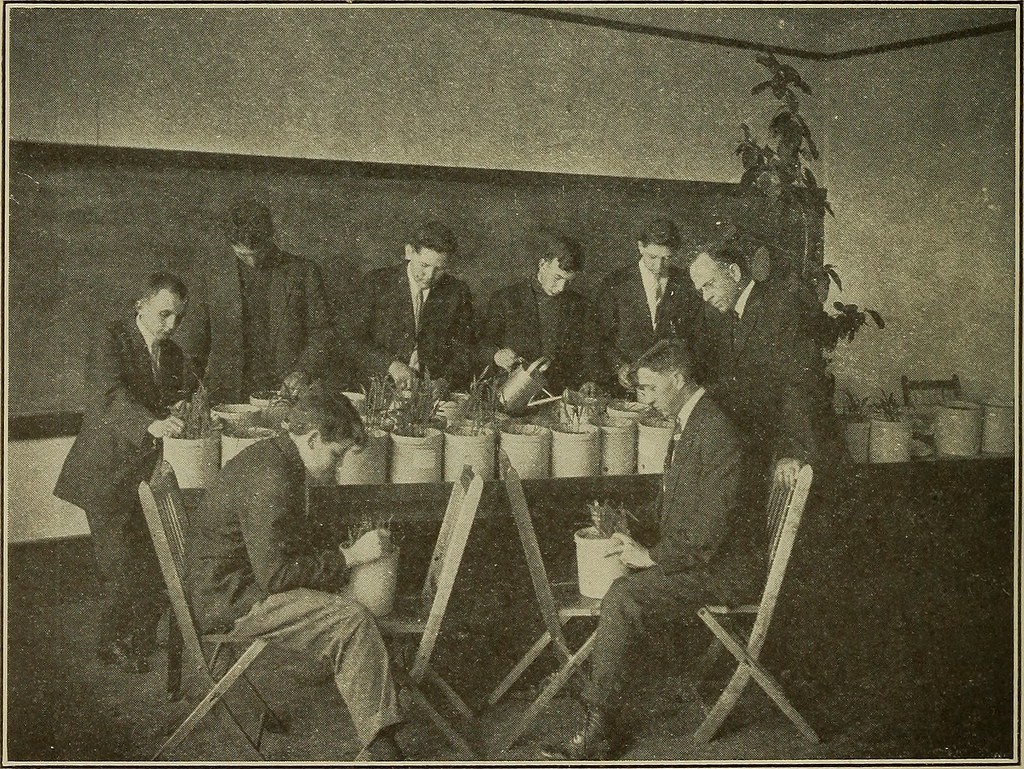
[934,399,1013,457]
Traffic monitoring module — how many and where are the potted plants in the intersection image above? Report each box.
[337,426,388,484]
[574,499,633,601]
[209,388,553,428]
[338,511,400,618]
[387,368,443,483]
[599,417,636,476]
[638,406,674,474]
[833,386,870,465]
[497,422,549,480]
[557,389,600,422]
[605,397,650,420]
[162,372,219,490]
[444,425,495,483]
[869,387,913,464]
[220,416,277,468]
[550,404,600,478]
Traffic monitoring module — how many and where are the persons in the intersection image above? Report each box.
[180,392,408,761]
[52,272,190,676]
[687,242,853,484]
[473,235,590,400]
[181,199,337,404]
[586,218,701,403]
[340,220,474,394]
[535,338,769,767]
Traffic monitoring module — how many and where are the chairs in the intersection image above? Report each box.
[346,465,484,758]
[484,460,602,750]
[136,460,287,760]
[901,373,960,405]
[695,457,821,745]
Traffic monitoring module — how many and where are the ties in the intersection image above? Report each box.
[671,416,681,465]
[414,286,428,349]
[733,311,739,345]
[653,272,664,324]
[150,339,161,386]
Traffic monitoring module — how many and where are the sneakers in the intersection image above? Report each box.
[537,700,620,759]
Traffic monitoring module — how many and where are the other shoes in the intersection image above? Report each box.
[100,643,149,673]
[133,623,156,653]
